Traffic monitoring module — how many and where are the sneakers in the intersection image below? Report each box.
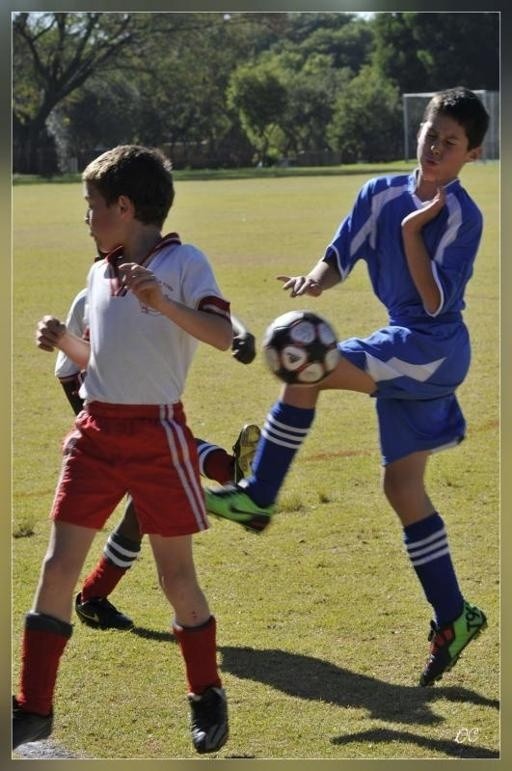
[419,600,488,687]
[203,484,274,535]
[232,424,262,483]
[187,687,229,755]
[75,592,133,629]
[12,695,54,750]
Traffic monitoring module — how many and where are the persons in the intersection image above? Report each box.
[201,85,491,689]
[55,243,260,631]
[13,144,230,754]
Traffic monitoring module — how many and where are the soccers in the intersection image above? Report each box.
[263,310,341,387]
